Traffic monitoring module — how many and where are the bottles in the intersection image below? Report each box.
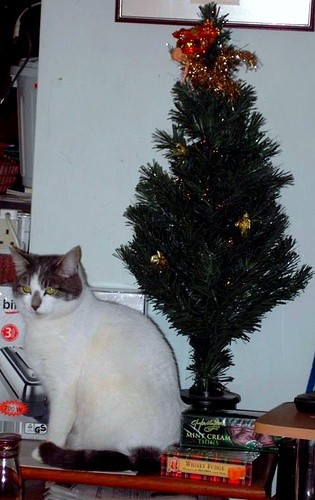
[0,432,23,500]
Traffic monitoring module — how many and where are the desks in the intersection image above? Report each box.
[255,403,315,500]
[17,438,280,500]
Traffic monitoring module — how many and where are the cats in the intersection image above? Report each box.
[10,246,184,475]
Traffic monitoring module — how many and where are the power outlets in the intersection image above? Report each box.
[0,218,18,249]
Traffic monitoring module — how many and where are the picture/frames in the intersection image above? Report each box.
[114,0,315,31]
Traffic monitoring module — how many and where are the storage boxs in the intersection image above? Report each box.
[160,409,286,486]
